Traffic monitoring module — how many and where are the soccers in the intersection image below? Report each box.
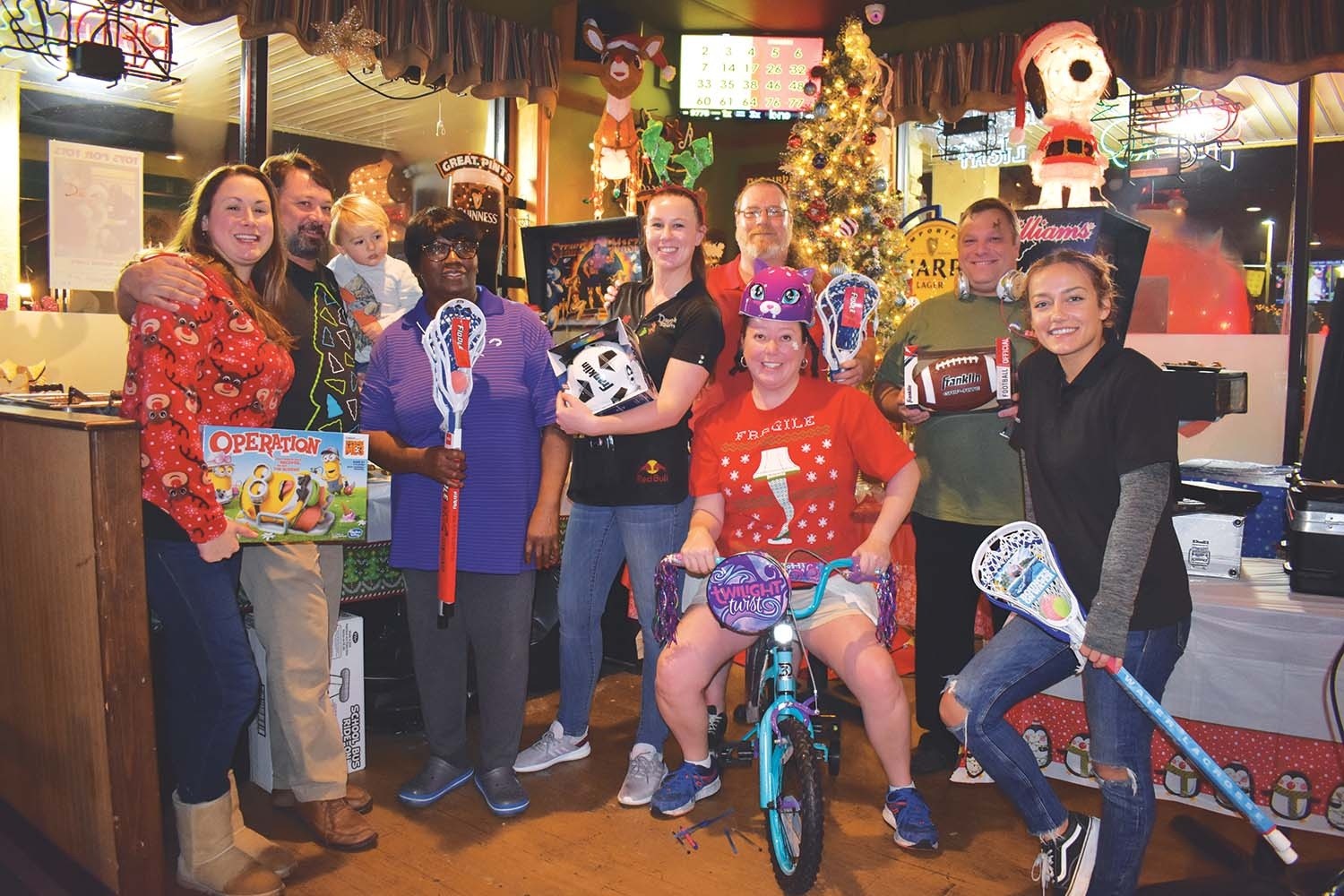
[563,340,641,413]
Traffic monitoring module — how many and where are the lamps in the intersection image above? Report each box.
[55,44,133,91]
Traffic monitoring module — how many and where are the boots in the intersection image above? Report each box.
[228,768,300,881]
[172,786,286,896]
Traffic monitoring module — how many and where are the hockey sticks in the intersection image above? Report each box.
[972,519,1301,865]
[816,268,881,385]
[420,298,490,633]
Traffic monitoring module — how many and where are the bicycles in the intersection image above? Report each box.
[661,550,887,892]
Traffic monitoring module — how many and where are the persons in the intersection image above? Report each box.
[358,202,572,817]
[509,182,725,808]
[873,197,1046,781]
[649,266,942,852]
[603,176,877,766]
[116,150,386,851]
[325,191,425,394]
[119,163,295,896]
[938,245,1197,896]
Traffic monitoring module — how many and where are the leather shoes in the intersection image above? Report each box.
[270,780,374,814]
[294,797,379,851]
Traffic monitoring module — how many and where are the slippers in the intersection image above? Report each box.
[398,754,475,804]
[474,766,530,816]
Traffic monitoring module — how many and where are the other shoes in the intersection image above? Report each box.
[733,698,773,724]
[906,732,959,775]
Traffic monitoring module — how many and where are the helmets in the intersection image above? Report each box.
[737,258,816,328]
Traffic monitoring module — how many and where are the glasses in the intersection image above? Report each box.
[420,238,479,262]
[735,206,793,218]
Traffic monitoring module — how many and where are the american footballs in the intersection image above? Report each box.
[912,350,998,414]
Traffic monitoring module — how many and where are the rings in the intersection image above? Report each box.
[875,568,882,575]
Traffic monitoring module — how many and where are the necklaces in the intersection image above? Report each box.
[1308,267,1328,300]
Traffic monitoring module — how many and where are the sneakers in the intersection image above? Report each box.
[882,787,940,851]
[512,720,592,773]
[1042,810,1100,896]
[706,705,728,756]
[617,742,670,807]
[650,754,722,818]
